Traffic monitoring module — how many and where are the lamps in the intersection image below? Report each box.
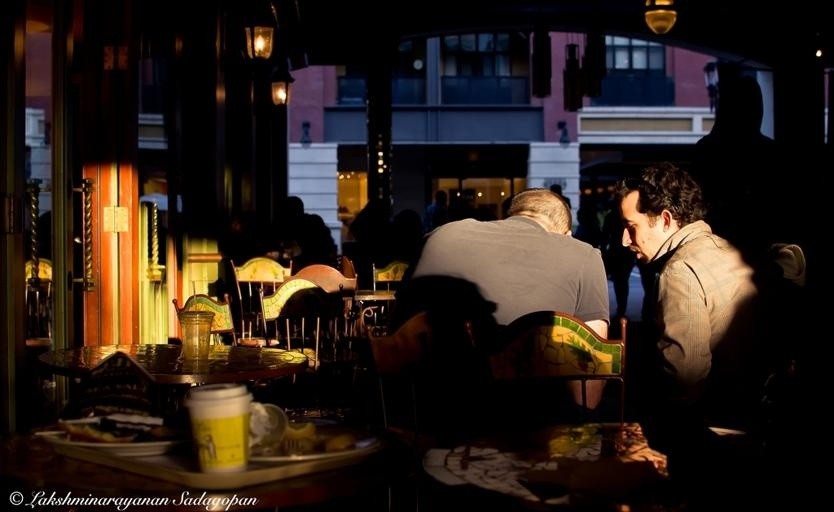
[263,63,294,110]
[240,23,279,67]
[641,1,678,40]
[700,60,726,113]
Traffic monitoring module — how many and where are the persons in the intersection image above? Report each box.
[228,67,832,511]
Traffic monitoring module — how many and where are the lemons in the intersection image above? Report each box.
[286,422,315,440]
[325,434,356,451]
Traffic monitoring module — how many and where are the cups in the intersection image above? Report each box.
[180,310,214,363]
[185,381,253,474]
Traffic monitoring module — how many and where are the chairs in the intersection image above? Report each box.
[1,256,667,512]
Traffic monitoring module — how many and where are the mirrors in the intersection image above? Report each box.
[13,9,70,467]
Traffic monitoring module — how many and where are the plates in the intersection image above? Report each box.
[247,436,382,464]
[33,427,191,459]
